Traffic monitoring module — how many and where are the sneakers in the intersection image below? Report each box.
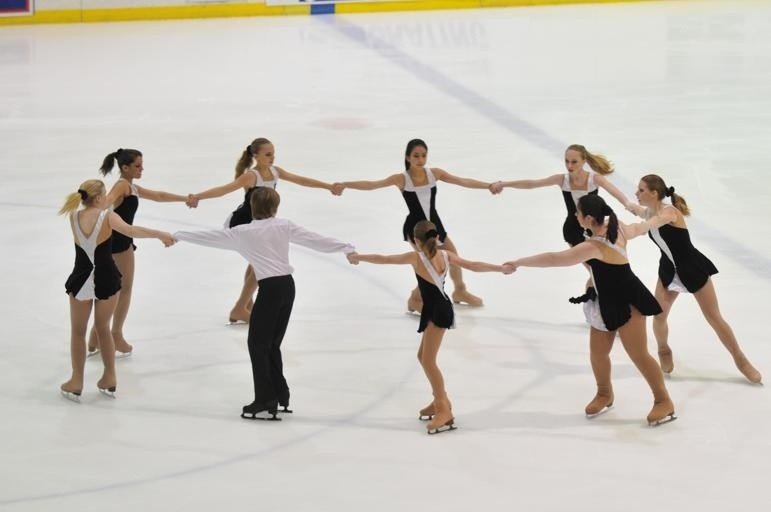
[88,335,98,352]
[426,398,452,430]
[408,290,423,314]
[419,393,453,415]
[229,302,251,323]
[452,287,482,306]
[736,355,761,383]
[646,391,674,421]
[97,372,117,392]
[242,396,278,412]
[658,349,674,373]
[585,383,614,415]
[61,378,82,397]
[111,333,132,353]
[277,393,289,406]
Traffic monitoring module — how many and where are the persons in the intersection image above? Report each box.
[494,144,637,294]
[165,186,358,421]
[86,149,199,359]
[628,173,764,386]
[335,139,502,317]
[58,180,177,403]
[503,195,677,428]
[186,138,343,327]
[351,222,513,435]
